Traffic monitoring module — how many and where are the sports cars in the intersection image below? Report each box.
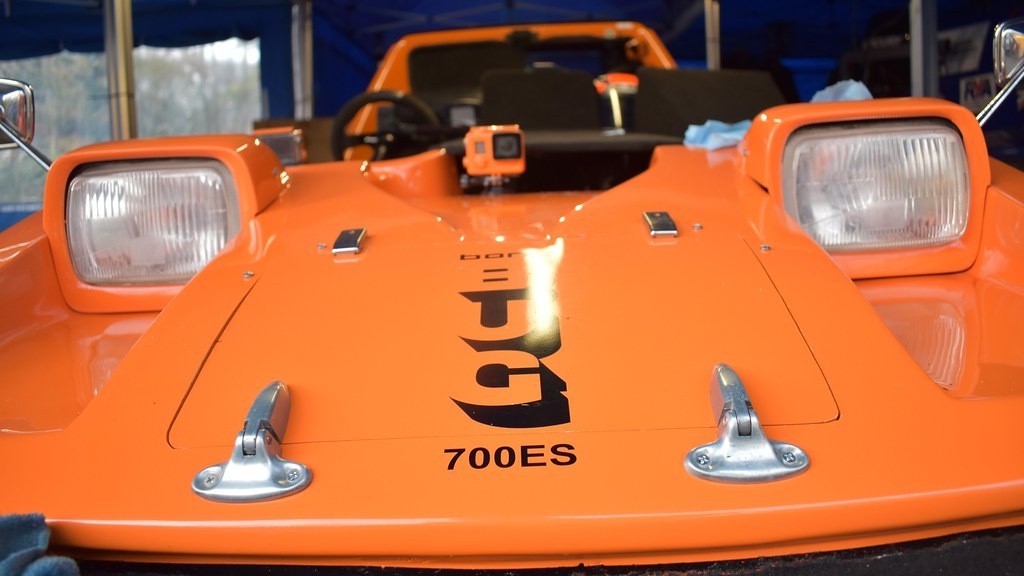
[0,23,1024,576]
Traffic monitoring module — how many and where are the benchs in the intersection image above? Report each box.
[438,62,788,161]
